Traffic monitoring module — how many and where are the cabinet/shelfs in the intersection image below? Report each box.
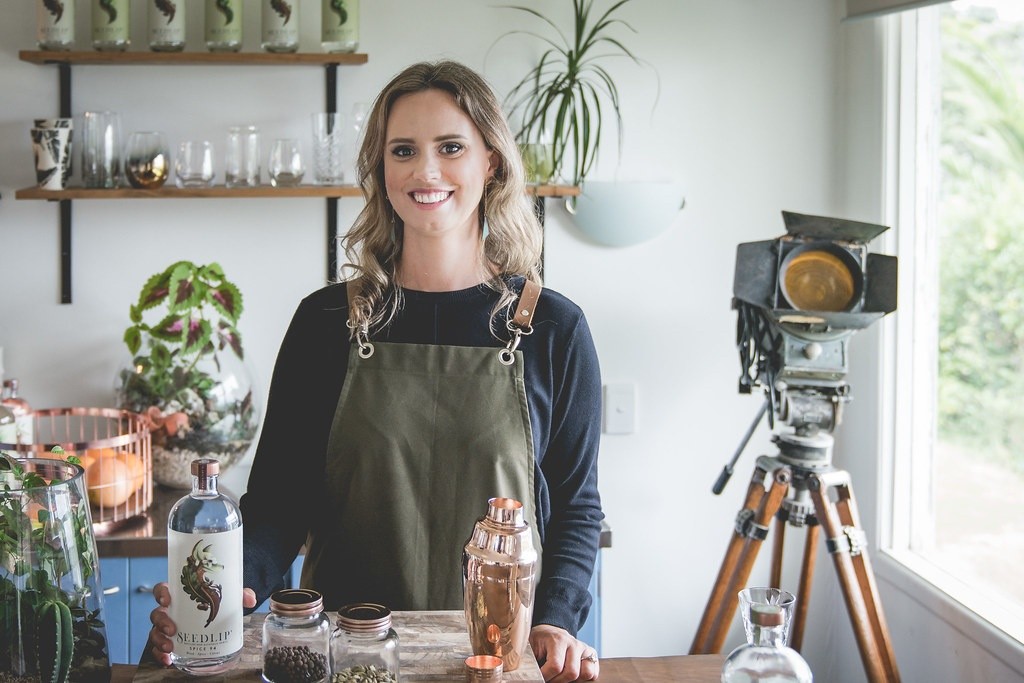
[13,48,581,199]
[0,557,288,666]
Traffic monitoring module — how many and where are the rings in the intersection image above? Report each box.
[582,653,598,663]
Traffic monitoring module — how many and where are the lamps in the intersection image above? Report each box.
[563,181,687,248]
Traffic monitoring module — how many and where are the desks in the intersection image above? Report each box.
[103,653,763,683]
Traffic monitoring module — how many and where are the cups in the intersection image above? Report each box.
[462,496,538,672]
[31,110,343,191]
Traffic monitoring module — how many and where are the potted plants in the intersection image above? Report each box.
[111,258,261,491]
[0,445,112,683]
[474,1,661,208]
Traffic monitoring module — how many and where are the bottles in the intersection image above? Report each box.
[329,602,399,683]
[167,458,243,676]
[261,589,331,683]
[721,587,813,683]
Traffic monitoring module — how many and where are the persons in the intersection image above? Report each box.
[149,61,606,683]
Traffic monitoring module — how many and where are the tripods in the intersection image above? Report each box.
[689,378,902,683]
